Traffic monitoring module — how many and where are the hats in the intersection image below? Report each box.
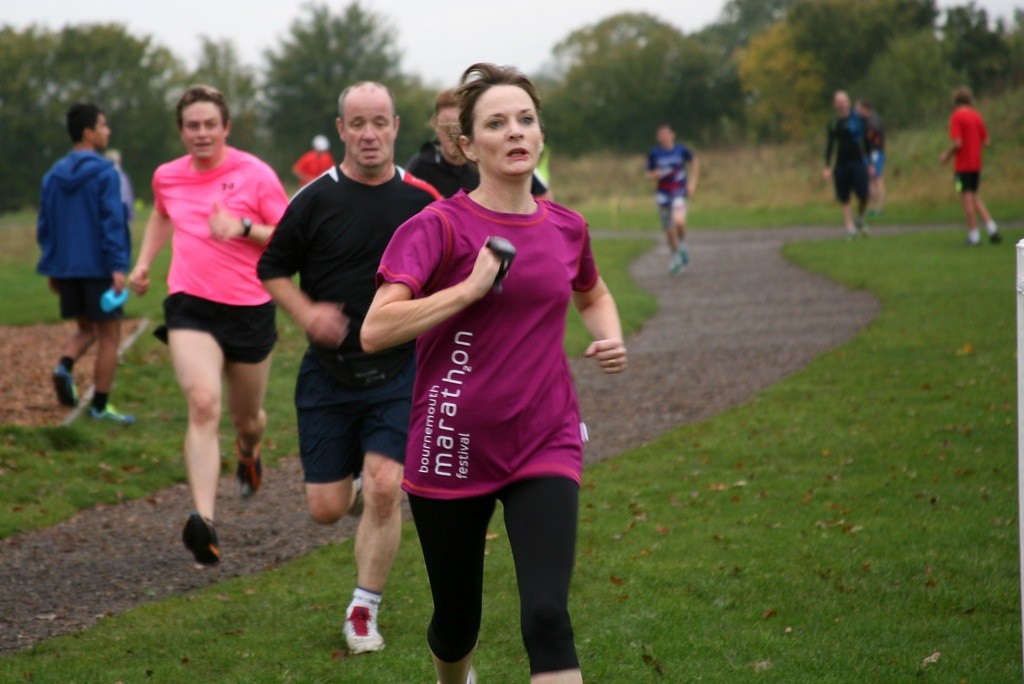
[313,135,329,152]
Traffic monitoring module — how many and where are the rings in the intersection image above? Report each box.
[609,359,616,367]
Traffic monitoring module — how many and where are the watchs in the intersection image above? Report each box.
[241,218,252,237]
[485,237,516,279]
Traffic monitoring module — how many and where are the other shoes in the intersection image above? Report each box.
[437,665,474,684]
[990,231,1003,245]
[678,245,688,265]
[855,221,871,237]
[666,263,681,275]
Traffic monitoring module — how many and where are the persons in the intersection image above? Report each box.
[129,86,289,568]
[941,87,1002,247]
[824,90,873,237]
[293,134,335,187]
[855,99,887,215]
[104,149,135,215]
[644,124,700,276]
[255,79,444,654]
[359,62,627,684]
[36,103,136,426]
[408,89,553,201]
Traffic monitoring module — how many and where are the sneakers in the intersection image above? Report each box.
[342,604,385,654]
[348,475,364,517]
[234,440,263,499]
[182,513,222,564]
[51,365,79,405]
[86,402,135,426]
